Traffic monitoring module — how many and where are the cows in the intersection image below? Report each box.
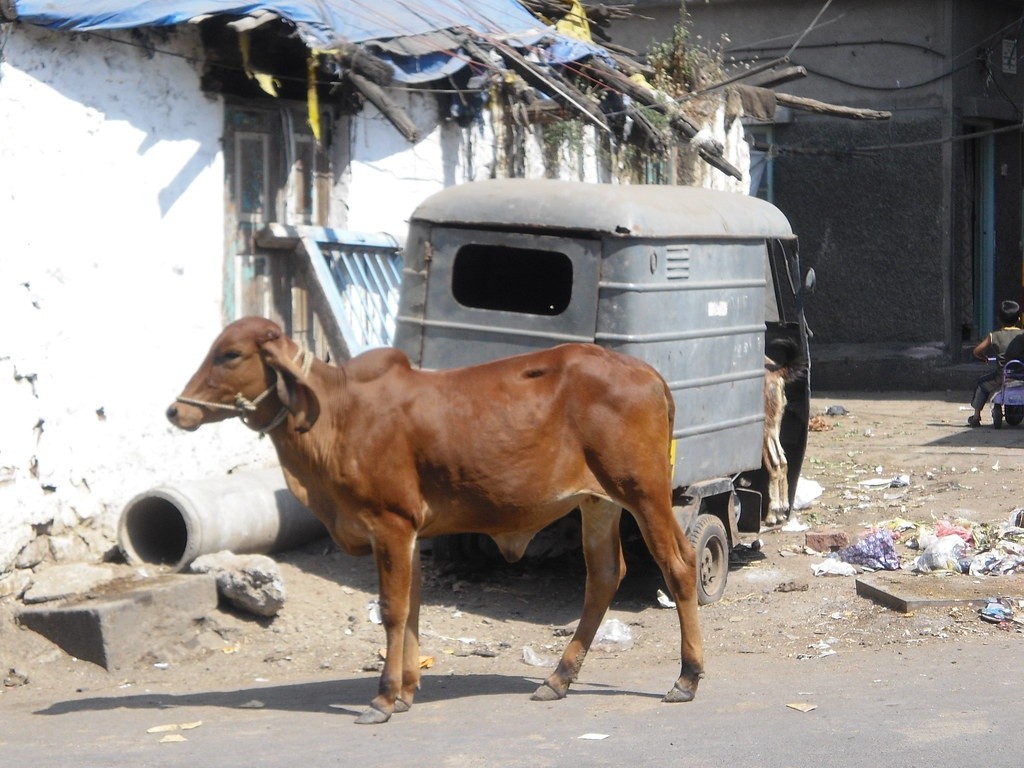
[167,317,704,727]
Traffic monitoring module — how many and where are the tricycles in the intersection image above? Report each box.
[983,357,1024,426]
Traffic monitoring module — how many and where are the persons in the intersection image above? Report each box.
[968,301,1024,427]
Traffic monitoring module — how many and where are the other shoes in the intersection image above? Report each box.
[968,416,981,428]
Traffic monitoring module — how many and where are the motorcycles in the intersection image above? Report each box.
[399,179,817,606]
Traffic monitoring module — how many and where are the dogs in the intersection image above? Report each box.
[762,334,807,527]
